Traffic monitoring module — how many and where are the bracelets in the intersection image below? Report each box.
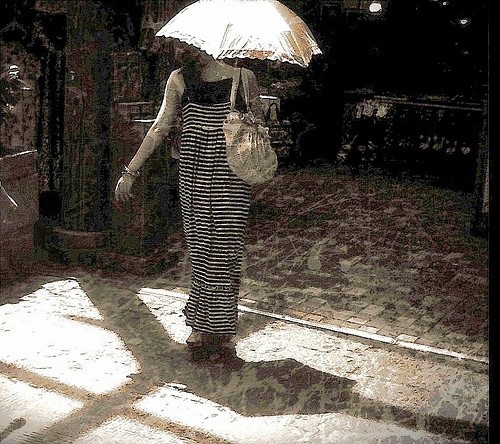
[121,166,140,178]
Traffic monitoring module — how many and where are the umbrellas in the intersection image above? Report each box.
[154,1,324,70]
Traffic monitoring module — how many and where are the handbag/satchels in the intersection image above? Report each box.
[223,111,278,184]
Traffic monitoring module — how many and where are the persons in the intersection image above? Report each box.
[116,35,278,348]
[266,102,279,121]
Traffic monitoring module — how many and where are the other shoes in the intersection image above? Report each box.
[186,331,228,350]
